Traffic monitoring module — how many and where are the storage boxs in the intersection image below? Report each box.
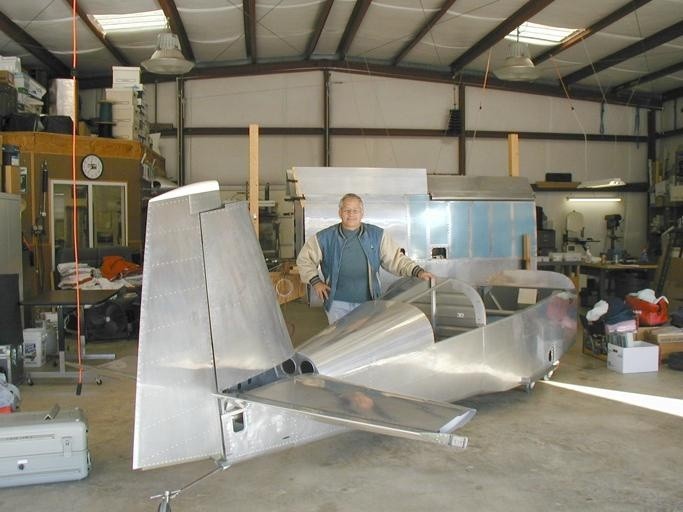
[648,339,683,363]
[667,182,683,202]
[104,65,154,149]
[0,55,48,131]
[606,339,660,374]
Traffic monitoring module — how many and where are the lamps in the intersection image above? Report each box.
[140,18,195,75]
[492,41,541,83]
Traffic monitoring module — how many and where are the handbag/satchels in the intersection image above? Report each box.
[595,296,633,324]
[624,289,668,327]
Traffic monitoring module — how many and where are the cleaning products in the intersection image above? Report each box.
[585,245,592,263]
[638,245,649,263]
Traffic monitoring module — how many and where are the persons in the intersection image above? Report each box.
[296,193,437,326]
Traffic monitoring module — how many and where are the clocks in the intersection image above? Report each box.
[81,154,104,180]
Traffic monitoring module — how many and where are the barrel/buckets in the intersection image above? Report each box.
[21,328,48,367]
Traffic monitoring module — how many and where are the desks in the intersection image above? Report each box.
[18,289,118,385]
[537,261,659,305]
